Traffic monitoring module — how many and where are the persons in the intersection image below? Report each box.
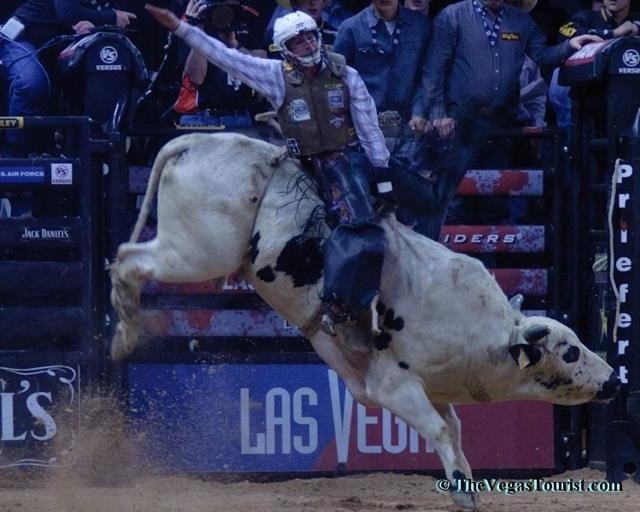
[0,0,640,332]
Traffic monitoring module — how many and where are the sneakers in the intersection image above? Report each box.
[322,310,373,355]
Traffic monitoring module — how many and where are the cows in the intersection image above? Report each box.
[105,128,624,511]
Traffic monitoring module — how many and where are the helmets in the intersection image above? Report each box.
[272,11,325,71]
